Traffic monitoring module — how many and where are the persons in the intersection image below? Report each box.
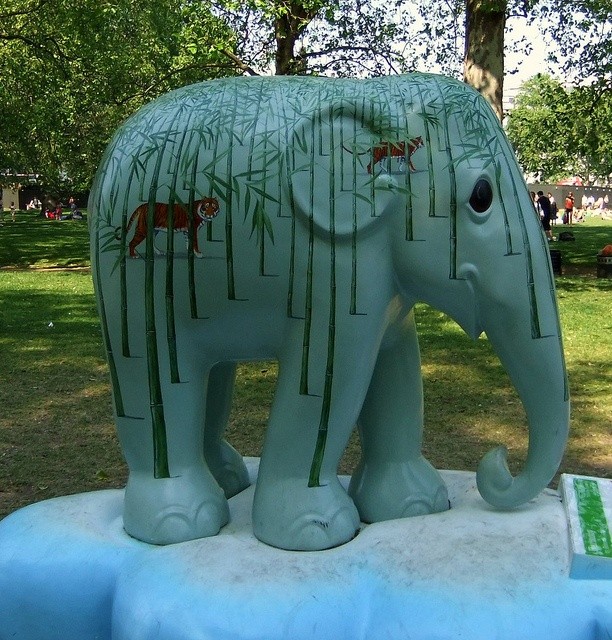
[564,192,575,228]
[529,192,542,213]
[537,191,553,241]
[573,194,612,222]
[9,202,16,222]
[546,192,558,226]
[26,195,84,220]
